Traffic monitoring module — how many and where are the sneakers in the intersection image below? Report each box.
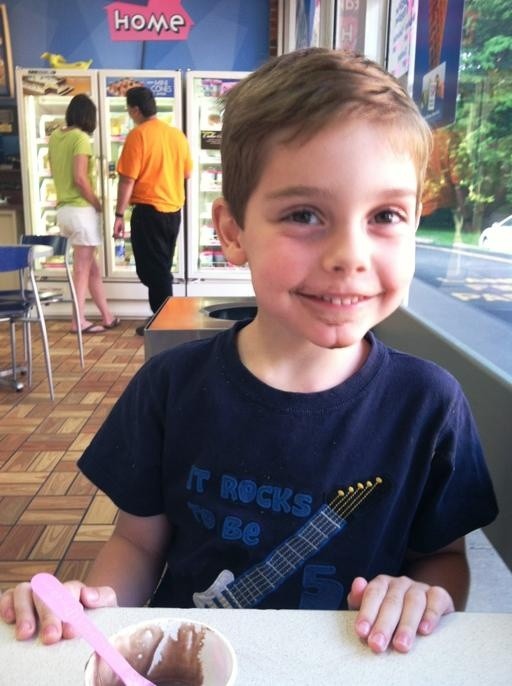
[135,316,157,338]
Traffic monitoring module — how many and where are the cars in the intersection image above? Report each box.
[476,212,512,256]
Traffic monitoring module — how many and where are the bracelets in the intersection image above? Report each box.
[115,213,123,217]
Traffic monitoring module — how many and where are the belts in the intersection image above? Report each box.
[132,202,153,209]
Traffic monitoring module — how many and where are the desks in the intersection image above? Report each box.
[142,297,259,365]
[0,606,511,686]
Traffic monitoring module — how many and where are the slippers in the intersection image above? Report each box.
[70,321,105,335]
[103,316,123,329]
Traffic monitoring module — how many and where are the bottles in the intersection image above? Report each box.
[113,233,126,264]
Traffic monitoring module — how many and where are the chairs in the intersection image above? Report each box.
[0,245,56,401]
[0,234,84,382]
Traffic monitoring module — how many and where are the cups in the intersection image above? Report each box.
[78,617,237,686]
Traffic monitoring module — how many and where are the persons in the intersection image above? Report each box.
[48,94,120,334]
[113,87,193,336]
[1,50,499,656]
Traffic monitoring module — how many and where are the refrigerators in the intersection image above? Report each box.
[14,70,184,301]
[185,68,268,297]
